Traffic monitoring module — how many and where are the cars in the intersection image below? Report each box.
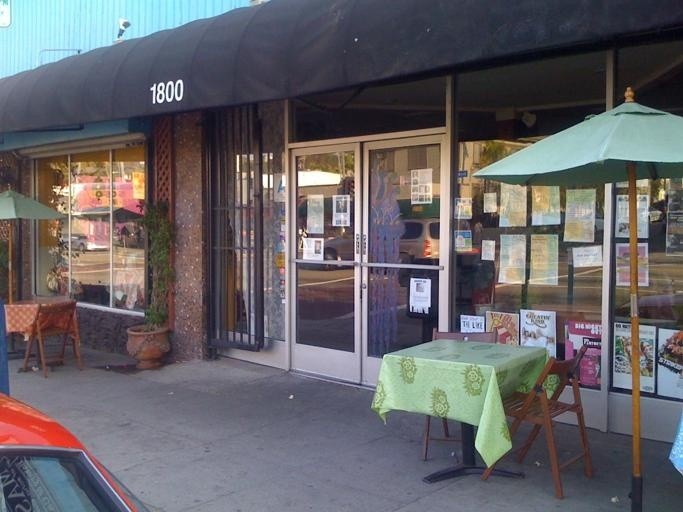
[0,386,150,512]
[64,234,109,251]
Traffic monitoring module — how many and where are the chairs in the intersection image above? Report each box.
[421,326,592,499]
[20,299,76,377]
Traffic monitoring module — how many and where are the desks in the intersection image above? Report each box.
[369,338,546,485]
[2,298,85,371]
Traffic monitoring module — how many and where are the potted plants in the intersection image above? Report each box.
[124,197,177,370]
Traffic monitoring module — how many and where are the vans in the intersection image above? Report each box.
[325,219,442,265]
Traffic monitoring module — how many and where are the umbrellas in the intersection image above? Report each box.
[470,86,683,512]
[76,203,145,229]
[0,183,68,351]
